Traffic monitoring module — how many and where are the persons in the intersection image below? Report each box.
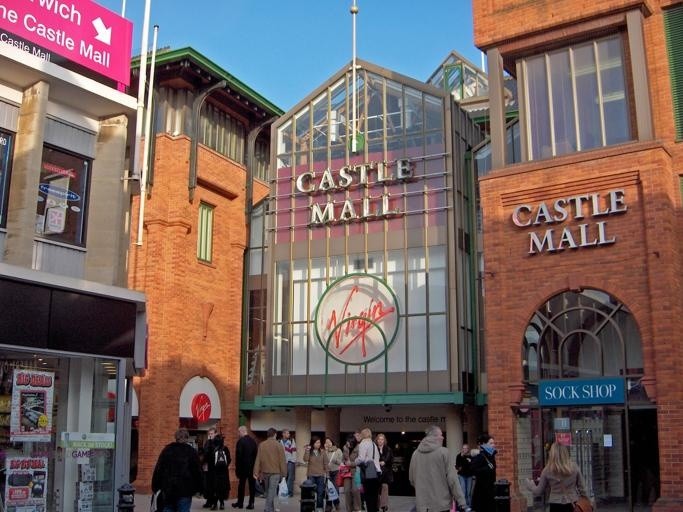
[470,435,499,511]
[456,442,474,507]
[524,439,595,512]
[301,427,391,512]
[205,433,231,510]
[232,424,258,509]
[278,429,297,497]
[254,427,286,512]
[408,424,472,512]
[198,427,219,508]
[150,427,203,512]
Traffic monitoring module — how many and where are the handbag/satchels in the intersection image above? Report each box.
[336,473,344,487]
[574,496,593,511]
[150,492,164,512]
[365,461,378,479]
[378,466,394,483]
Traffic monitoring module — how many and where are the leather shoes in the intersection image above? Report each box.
[204,502,254,510]
